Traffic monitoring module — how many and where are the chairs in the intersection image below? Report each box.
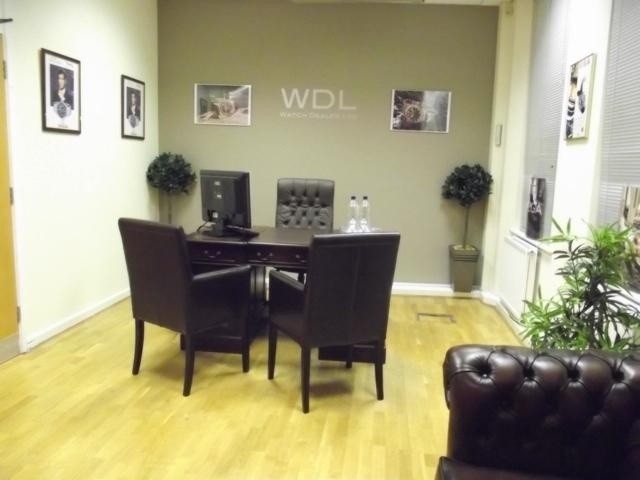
[267,232,400,414]
[274,178,334,284]
[118,218,252,396]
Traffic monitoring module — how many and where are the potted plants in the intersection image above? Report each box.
[440,162,493,292]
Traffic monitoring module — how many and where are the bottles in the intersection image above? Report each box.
[359,196,370,232]
[347,196,359,232]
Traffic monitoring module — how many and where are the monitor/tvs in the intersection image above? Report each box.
[199,170,250,237]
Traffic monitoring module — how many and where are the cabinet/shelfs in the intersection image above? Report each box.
[498,238,542,325]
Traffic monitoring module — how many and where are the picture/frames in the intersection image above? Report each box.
[121,75,146,140]
[42,48,81,133]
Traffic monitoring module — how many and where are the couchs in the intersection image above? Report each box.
[433,345,640,479]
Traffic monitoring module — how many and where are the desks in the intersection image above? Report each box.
[180,225,386,366]
